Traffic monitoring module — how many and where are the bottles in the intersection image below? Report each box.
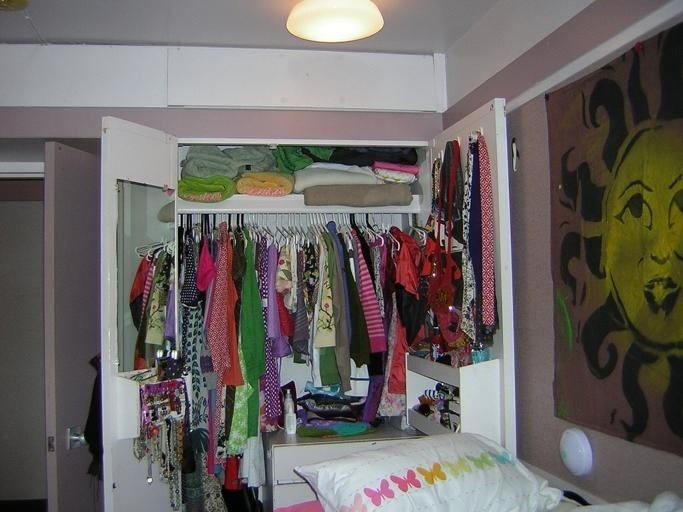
[285,405,297,434]
[284,389,294,427]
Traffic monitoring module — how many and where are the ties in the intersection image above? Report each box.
[460,134,499,350]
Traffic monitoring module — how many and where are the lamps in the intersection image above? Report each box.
[286,0,385,43]
[560,428,593,476]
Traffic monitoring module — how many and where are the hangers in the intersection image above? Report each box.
[135,210,427,262]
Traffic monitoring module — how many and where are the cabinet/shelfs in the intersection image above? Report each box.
[405,353,501,444]
[94,95,518,512]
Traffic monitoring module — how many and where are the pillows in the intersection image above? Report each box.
[293,433,564,512]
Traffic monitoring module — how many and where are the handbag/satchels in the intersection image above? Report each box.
[425,277,456,315]
